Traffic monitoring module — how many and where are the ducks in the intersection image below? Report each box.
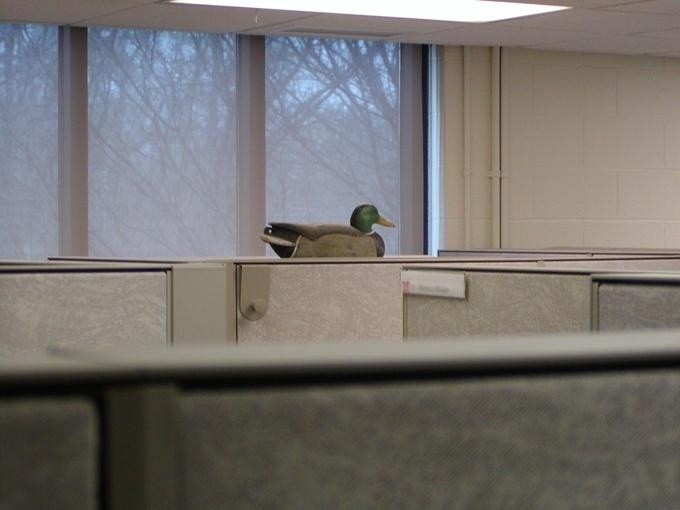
[260,204,395,258]
